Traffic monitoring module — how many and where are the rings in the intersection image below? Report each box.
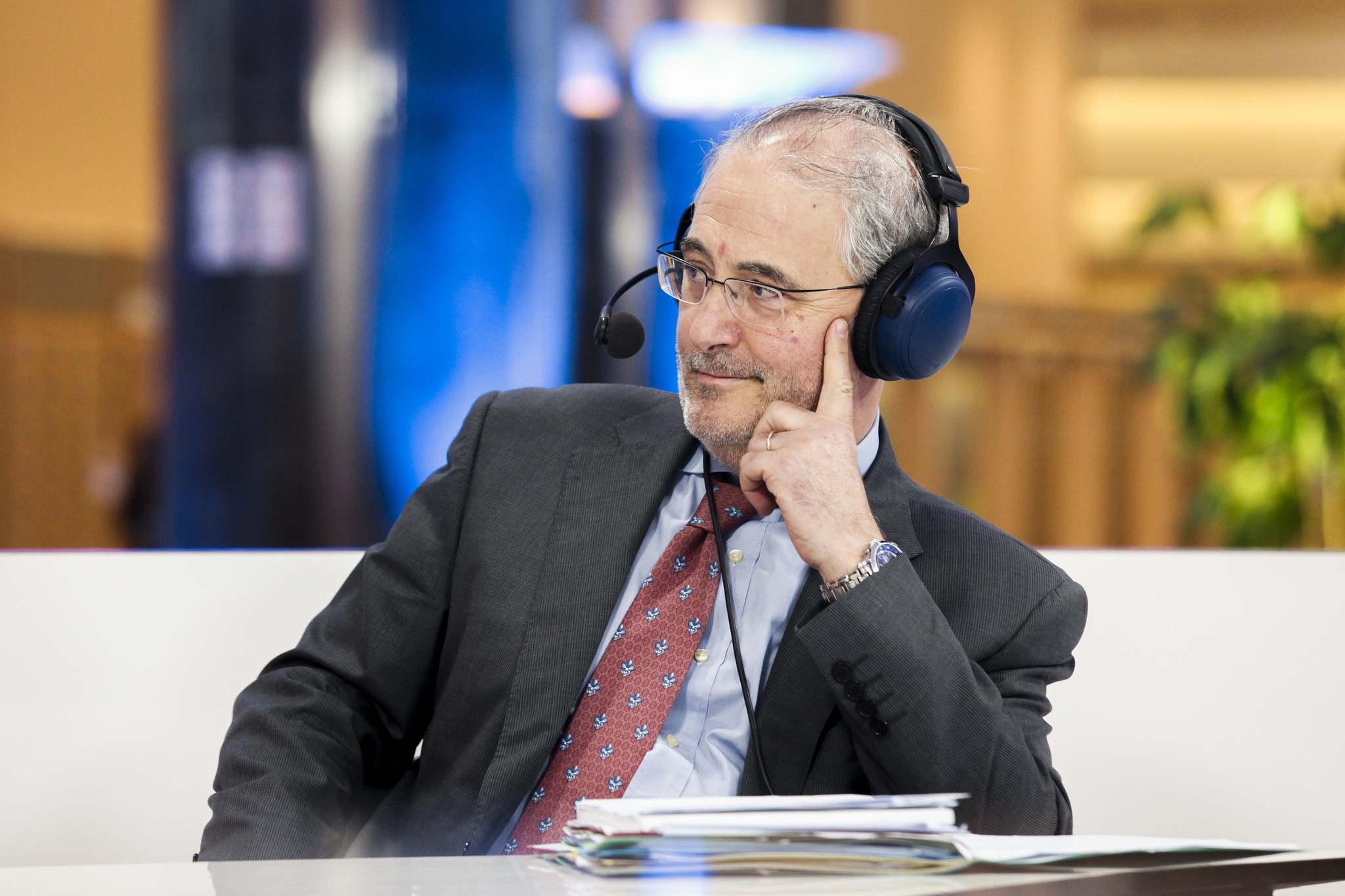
[766,430,779,452]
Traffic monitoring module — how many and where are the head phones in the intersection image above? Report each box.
[669,95,976,382]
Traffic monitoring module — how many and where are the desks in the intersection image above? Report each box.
[0,820,1345,894]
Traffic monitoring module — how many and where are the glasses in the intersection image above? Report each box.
[655,242,868,329]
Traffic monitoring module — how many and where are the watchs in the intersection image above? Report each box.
[817,538,903,606]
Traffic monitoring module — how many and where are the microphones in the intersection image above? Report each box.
[597,266,664,360]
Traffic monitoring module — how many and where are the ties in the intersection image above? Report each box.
[501,474,754,857]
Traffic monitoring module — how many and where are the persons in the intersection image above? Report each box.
[188,97,1088,863]
